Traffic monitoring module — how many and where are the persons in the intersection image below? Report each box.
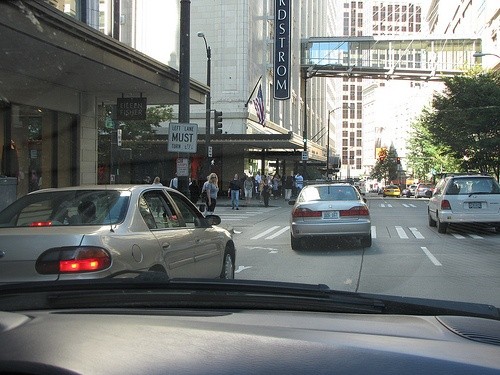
[149,176,164,217]
[241,170,304,209]
[228,173,242,210]
[169,172,178,190]
[78,201,99,224]
[188,173,219,214]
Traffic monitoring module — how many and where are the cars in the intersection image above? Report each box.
[425,175,500,236]
[408,185,418,196]
[0,183,236,293]
[288,184,372,250]
[415,183,435,199]
[383,185,401,198]
[378,188,384,194]
[402,188,409,196]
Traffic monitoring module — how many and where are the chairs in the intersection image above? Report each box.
[70,215,95,224]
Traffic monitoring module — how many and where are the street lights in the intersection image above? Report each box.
[195,31,214,206]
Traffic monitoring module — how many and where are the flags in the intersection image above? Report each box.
[252,83,266,127]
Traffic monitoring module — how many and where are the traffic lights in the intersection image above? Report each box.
[397,157,400,164]
